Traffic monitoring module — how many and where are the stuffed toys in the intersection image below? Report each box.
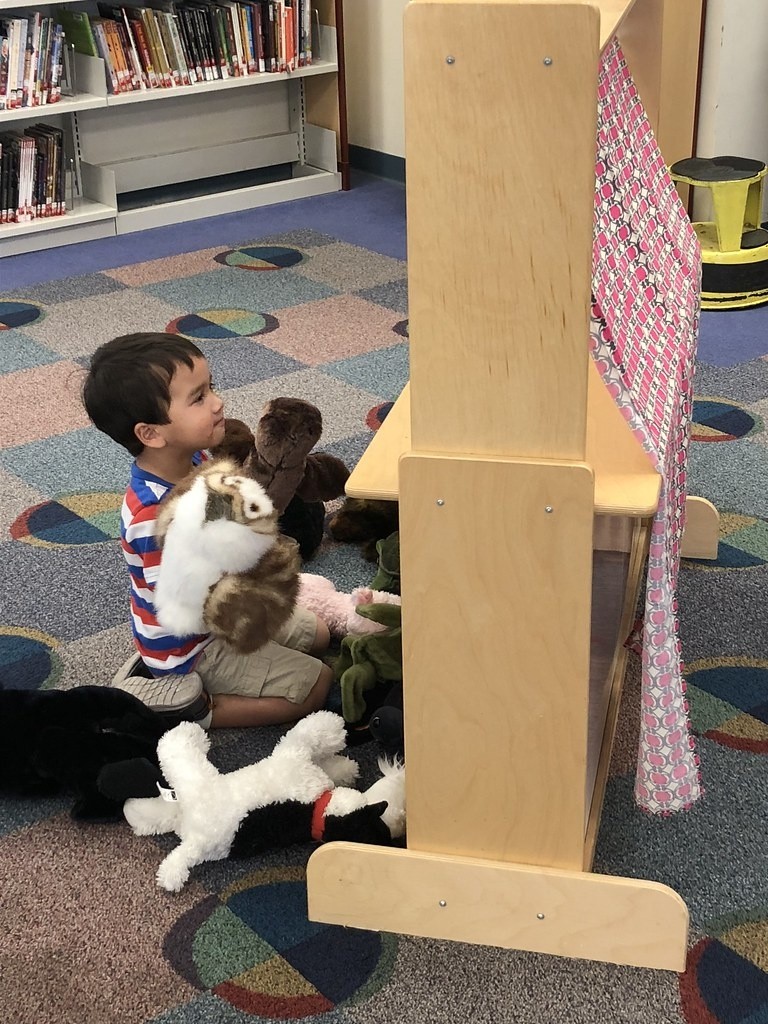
[0,395,404,893]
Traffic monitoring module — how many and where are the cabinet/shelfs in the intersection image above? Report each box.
[0,1,342,257]
[304,0,706,981]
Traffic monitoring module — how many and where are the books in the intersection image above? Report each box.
[0,0,326,225]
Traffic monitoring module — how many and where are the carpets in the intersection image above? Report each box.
[0,227,767,1024]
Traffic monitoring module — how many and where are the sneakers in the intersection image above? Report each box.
[110,650,156,689]
[113,671,212,728]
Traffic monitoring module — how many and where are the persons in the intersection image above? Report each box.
[83,333,336,731]
